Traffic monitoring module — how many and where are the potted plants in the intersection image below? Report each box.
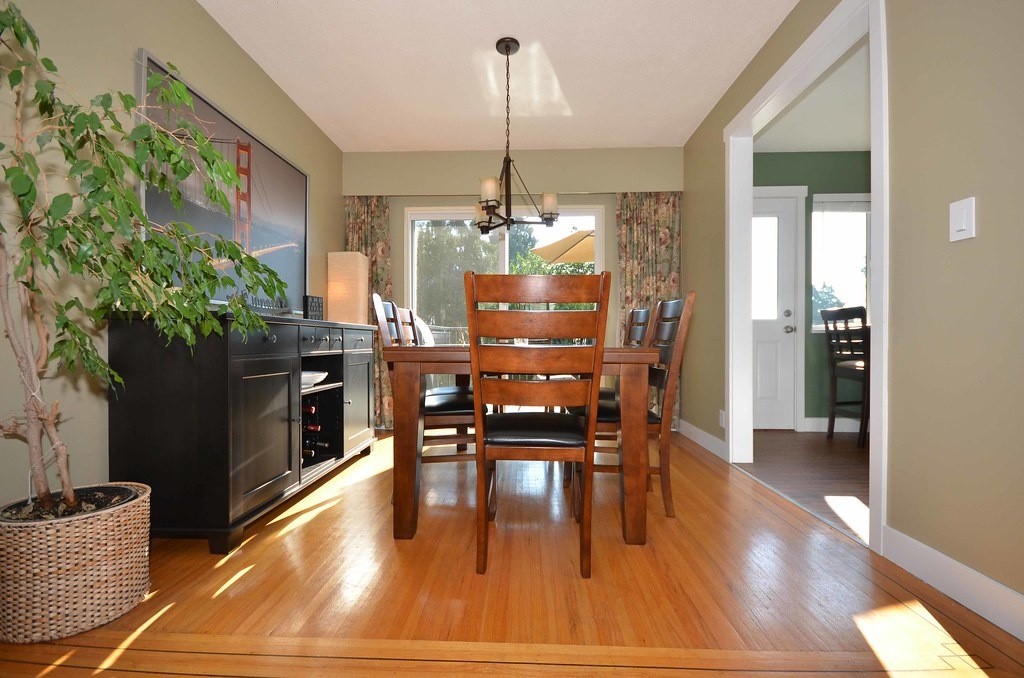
[0,1,288,645]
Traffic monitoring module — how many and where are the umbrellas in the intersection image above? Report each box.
[531,230,595,265]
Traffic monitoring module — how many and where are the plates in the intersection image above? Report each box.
[301,371,328,388]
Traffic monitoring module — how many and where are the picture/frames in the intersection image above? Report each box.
[133,48,311,316]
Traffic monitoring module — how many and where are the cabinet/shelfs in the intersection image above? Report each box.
[102,309,378,556]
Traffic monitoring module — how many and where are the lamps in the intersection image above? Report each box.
[474,38,561,237]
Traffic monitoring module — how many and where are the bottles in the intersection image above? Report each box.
[302,406,329,459]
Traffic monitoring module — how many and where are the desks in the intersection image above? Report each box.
[382,342,661,546]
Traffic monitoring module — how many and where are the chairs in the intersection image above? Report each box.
[372,293,489,505]
[563,305,651,487]
[819,305,872,447]
[397,308,479,396]
[463,269,610,578]
[561,289,698,526]
